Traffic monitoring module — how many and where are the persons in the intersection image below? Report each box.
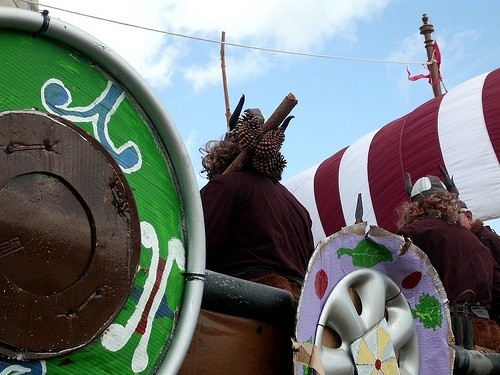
[393,157,499,333]
[184,94,323,298]
[448,199,500,325]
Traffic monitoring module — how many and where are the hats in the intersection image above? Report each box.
[403,163,458,201]
[456,200,468,214]
